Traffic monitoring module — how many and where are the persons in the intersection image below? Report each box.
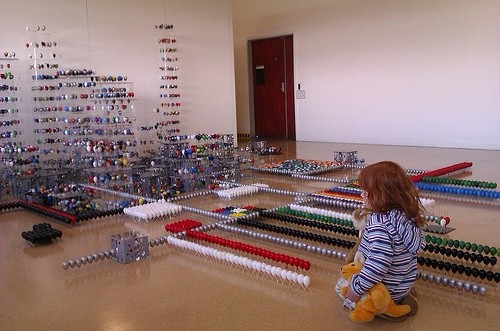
[344,161,428,322]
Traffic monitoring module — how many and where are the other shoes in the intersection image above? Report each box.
[397,294,419,317]
[377,302,407,322]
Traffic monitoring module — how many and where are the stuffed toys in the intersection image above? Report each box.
[344,208,375,265]
[339,252,411,322]
